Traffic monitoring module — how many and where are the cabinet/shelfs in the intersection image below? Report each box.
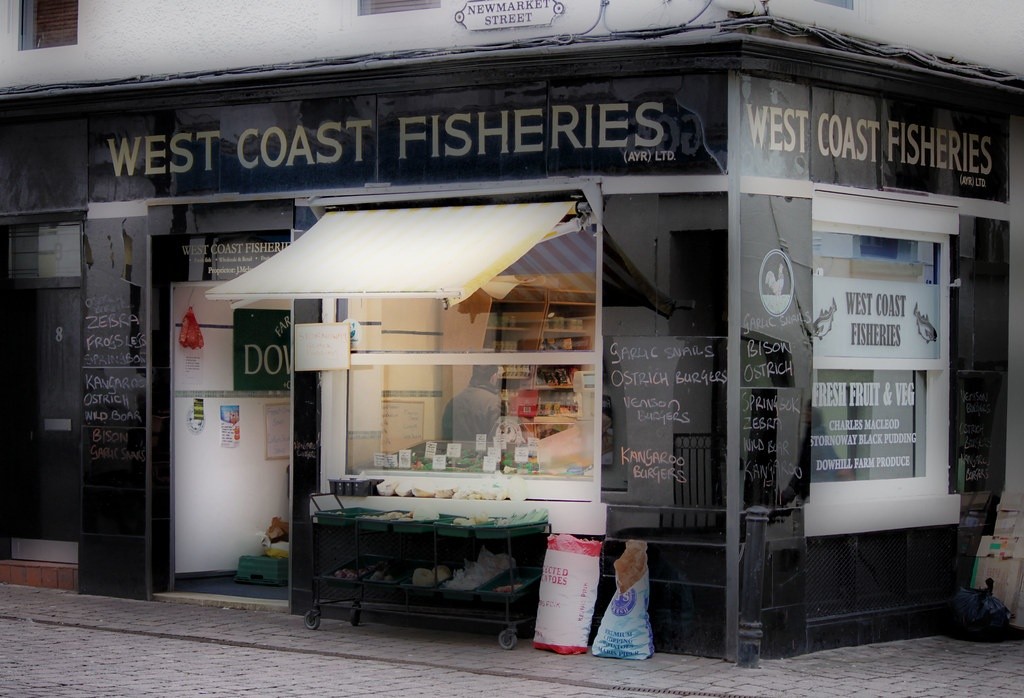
[443,292,594,429]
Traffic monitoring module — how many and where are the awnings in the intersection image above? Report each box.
[204,202,594,309]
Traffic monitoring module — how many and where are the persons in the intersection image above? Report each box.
[440,362,505,441]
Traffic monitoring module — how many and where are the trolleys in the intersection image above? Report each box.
[300,487,559,651]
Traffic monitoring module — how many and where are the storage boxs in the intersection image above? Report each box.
[320,504,550,541]
[972,487,1024,630]
